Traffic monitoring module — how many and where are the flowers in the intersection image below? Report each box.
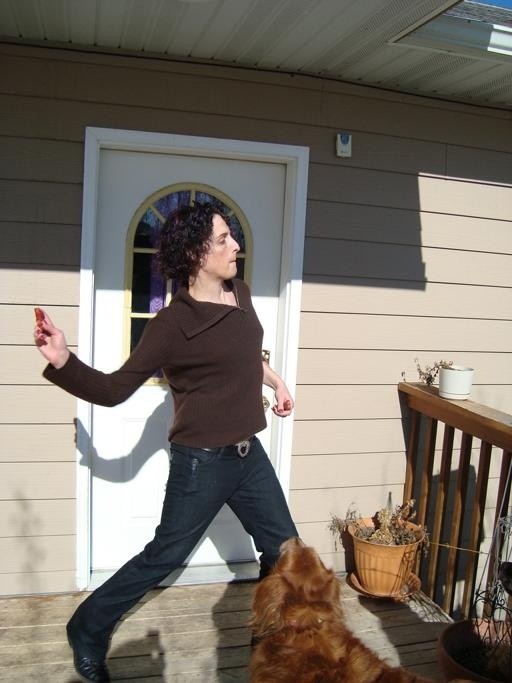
[401,356,456,389]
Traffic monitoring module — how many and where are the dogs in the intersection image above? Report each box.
[247,533,436,683]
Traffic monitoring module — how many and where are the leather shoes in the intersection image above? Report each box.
[65,622,112,682]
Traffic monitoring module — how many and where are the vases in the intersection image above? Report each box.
[348,517,427,594]
[438,617,511,683]
[438,364,475,401]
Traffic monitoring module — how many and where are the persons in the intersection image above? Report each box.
[33,200,301,682]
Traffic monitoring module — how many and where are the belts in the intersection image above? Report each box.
[201,436,253,457]
